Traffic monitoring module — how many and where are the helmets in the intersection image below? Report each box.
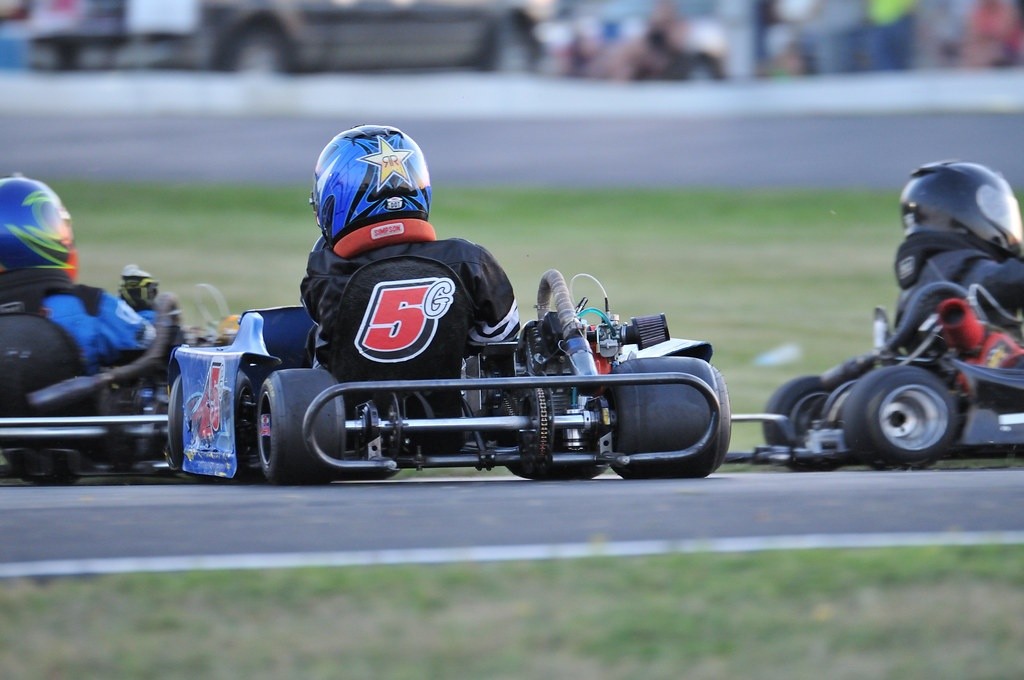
[309,124,431,248]
[900,158,1023,259]
[0,175,74,274]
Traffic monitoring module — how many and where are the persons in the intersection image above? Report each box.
[895,162,1023,332]
[1,177,157,377]
[301,124,520,374]
[637,27,690,81]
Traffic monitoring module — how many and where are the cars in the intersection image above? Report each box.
[28,0,548,72]
